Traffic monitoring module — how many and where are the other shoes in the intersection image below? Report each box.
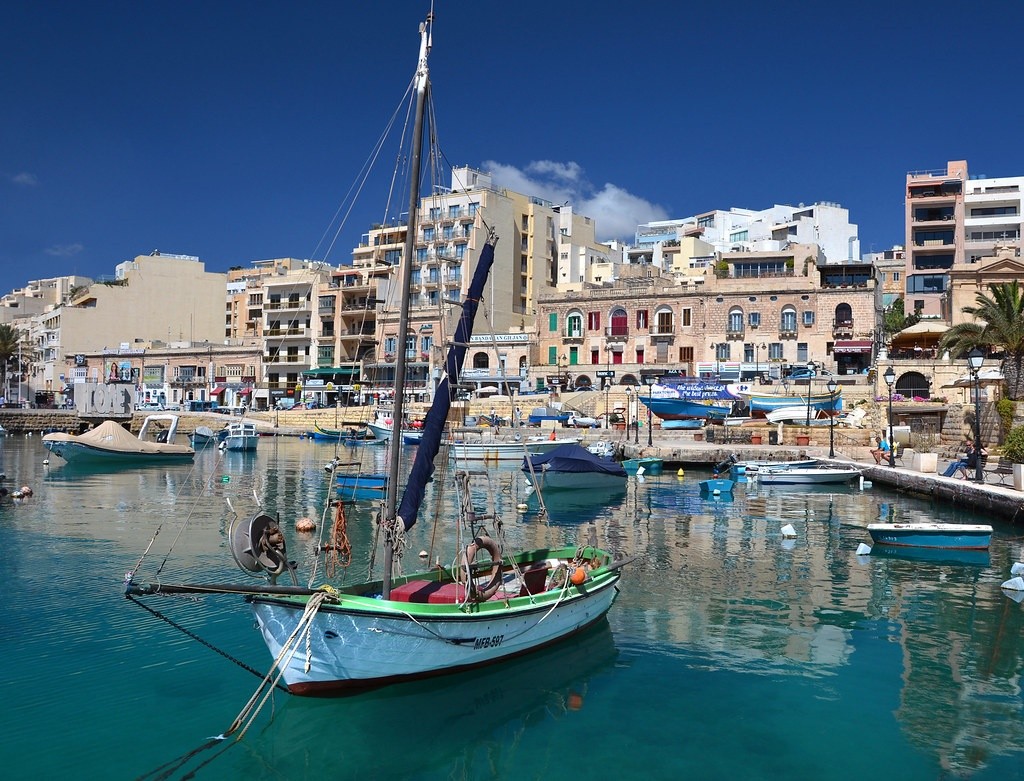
[938,471,943,475]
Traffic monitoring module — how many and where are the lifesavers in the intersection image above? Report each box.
[460,537,502,601]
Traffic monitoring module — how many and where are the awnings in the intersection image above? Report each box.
[833,341,873,353]
[241,387,252,395]
[209,387,226,395]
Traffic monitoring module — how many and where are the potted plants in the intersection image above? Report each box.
[830,285,836,288]
[841,281,847,288]
[716,260,729,278]
[1000,424,1024,490]
[859,281,867,287]
[852,283,857,288]
[912,423,939,473]
[822,281,829,289]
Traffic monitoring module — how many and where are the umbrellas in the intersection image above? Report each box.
[890,320,952,359]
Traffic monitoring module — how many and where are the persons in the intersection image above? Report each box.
[354,393,359,406]
[870,437,889,465]
[495,415,499,433]
[883,435,897,463]
[632,415,636,429]
[939,438,988,480]
[490,407,495,419]
[62,397,72,410]
[516,407,523,426]
[109,362,121,380]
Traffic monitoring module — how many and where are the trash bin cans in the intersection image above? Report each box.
[707,430,714,442]
[26,401,32,409]
[769,431,778,445]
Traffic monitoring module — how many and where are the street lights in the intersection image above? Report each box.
[635,381,644,445]
[806,360,816,429]
[275,395,280,428]
[333,396,339,429]
[825,377,840,460]
[646,373,655,447]
[625,386,632,444]
[603,384,613,430]
[710,341,727,379]
[463,387,468,426]
[603,344,614,386]
[751,341,767,382]
[967,344,990,485]
[509,383,518,428]
[882,366,903,469]
[551,353,568,385]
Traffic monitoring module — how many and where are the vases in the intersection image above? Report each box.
[694,434,704,441]
[612,422,626,430]
[653,424,661,430]
[794,436,810,446]
[751,436,762,445]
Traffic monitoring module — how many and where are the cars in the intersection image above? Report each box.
[136,401,164,412]
[564,410,584,427]
[785,369,816,379]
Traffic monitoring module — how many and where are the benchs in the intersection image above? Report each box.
[893,446,904,467]
[982,456,1015,487]
[713,429,752,445]
[953,454,988,481]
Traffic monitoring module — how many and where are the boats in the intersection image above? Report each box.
[43,412,200,463]
[188,424,217,446]
[638,386,850,432]
[864,517,994,548]
[306,395,616,469]
[223,420,262,452]
[524,444,636,496]
[699,472,736,497]
[865,541,993,570]
[715,452,820,476]
[620,456,665,478]
[324,455,397,488]
[742,461,873,484]
[336,487,389,503]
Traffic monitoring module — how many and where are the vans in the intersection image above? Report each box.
[533,406,562,417]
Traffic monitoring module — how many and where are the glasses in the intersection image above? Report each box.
[967,444,971,446]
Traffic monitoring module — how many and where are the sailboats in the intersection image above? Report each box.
[116,6,629,700]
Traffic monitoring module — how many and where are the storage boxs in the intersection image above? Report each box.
[555,420,563,428]
[389,580,519,604]
[541,420,555,429]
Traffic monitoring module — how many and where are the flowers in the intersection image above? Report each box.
[384,352,396,363]
[421,352,429,359]
